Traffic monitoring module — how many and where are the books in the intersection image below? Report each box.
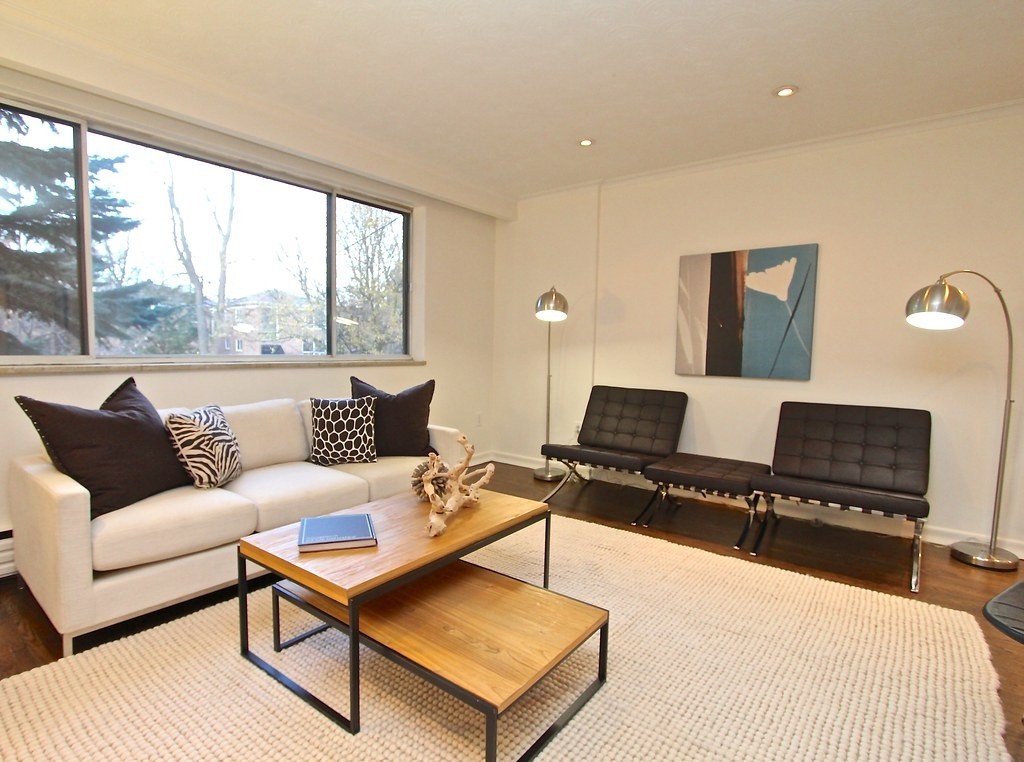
[298,514,378,552]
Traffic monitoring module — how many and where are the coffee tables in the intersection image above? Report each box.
[237,477,618,762]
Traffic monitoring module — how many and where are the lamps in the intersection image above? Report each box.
[530,285,569,482]
[903,270,1020,572]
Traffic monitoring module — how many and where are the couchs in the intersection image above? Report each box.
[4,397,462,656]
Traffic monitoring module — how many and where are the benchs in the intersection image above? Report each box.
[541,384,931,593]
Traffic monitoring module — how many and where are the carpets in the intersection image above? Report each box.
[0,510,1016,762]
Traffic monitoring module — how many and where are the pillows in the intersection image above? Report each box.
[9,376,196,520]
[349,375,439,457]
[163,403,244,490]
[306,395,378,467]
